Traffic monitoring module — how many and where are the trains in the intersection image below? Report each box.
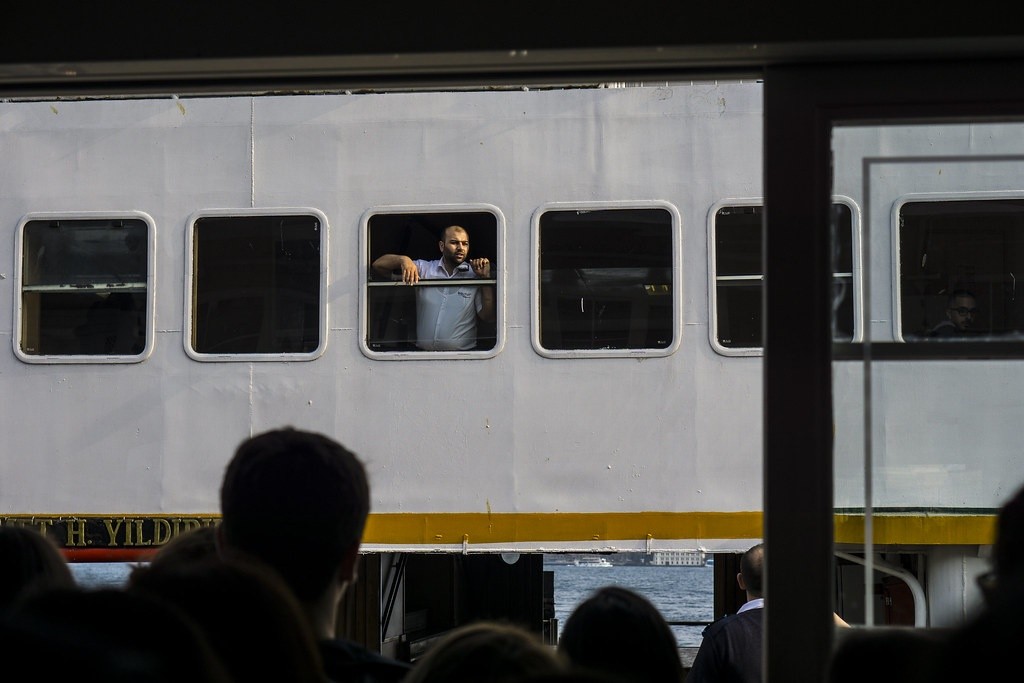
[1,33,1024,553]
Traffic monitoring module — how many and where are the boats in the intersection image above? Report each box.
[576,555,613,568]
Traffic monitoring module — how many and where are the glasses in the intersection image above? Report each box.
[948,306,977,317]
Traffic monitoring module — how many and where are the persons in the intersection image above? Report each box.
[923,289,978,339]
[372,226,494,351]
[0,426,1024,683]
